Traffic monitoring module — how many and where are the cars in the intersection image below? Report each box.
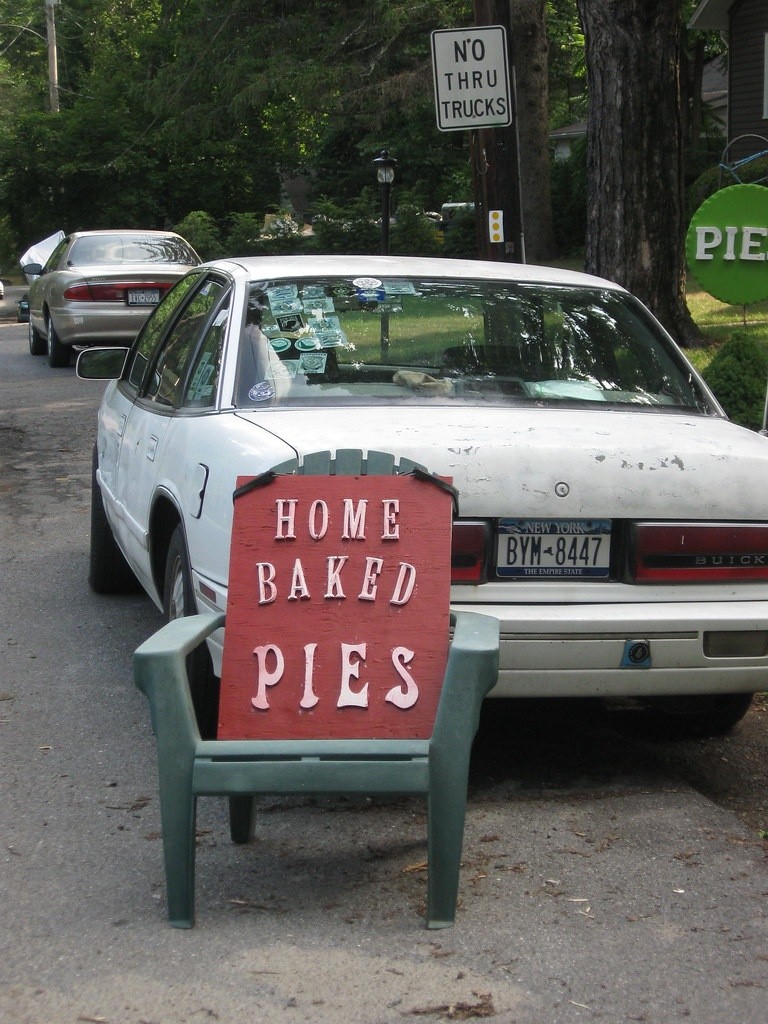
[14,293,29,323]
[0,277,5,299]
[90,257,768,731]
[22,229,206,368]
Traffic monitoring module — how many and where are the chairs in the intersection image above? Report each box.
[438,344,552,381]
[264,337,337,385]
[130,445,504,934]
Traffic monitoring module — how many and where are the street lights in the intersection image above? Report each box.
[371,148,399,366]
